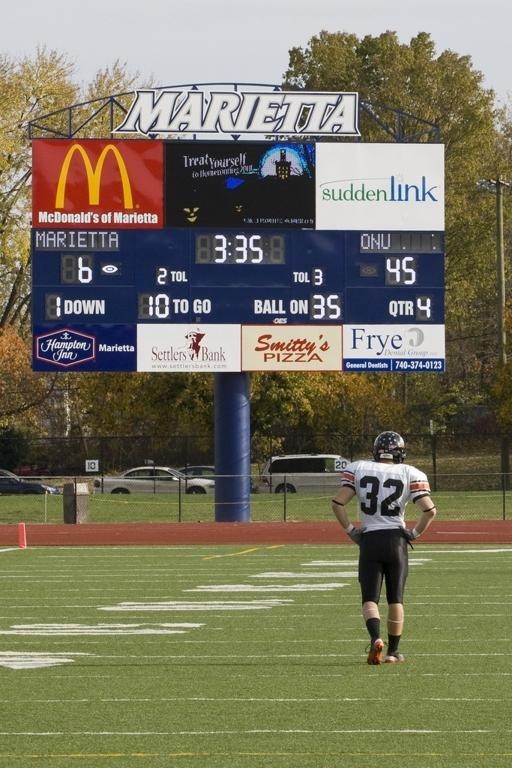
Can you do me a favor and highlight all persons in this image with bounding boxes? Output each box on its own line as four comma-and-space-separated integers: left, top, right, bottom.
332, 430, 436, 665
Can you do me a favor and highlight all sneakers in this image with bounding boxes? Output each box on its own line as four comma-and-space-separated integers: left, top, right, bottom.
384, 651, 405, 663
365, 638, 384, 665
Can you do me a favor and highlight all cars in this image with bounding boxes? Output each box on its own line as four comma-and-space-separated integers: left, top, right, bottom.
178, 467, 258, 492
0, 470, 61, 494
94, 464, 218, 495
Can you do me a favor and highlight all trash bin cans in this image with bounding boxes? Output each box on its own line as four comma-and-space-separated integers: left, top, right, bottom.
63, 483, 90, 524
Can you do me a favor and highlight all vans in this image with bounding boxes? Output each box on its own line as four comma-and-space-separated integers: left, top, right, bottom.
263, 451, 352, 493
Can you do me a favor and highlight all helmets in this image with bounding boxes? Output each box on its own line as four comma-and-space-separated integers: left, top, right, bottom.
373, 431, 406, 464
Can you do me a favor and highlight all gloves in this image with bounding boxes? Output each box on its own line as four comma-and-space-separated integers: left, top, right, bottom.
399, 525, 416, 542
347, 527, 367, 544
